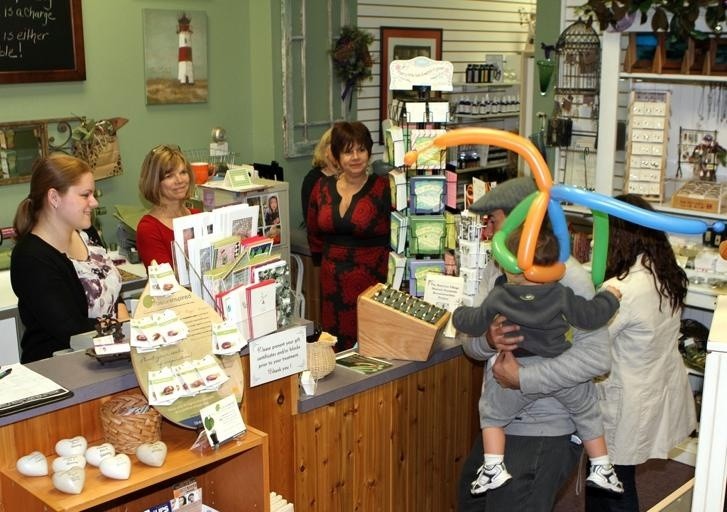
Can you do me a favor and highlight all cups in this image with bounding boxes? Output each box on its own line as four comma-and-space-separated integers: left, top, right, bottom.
190, 162, 216, 184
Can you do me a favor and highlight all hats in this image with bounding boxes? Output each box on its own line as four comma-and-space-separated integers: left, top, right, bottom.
466, 170, 540, 215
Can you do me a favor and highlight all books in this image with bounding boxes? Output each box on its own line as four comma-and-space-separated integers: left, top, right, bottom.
385, 99, 460, 298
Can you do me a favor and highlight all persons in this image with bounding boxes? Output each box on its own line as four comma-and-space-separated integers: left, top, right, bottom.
178, 495, 186, 506
306, 121, 392, 341
9, 154, 131, 364
450, 224, 625, 494
298, 126, 341, 268
264, 197, 280, 225
583, 193, 698, 512
135, 143, 204, 272
456, 176, 613, 511
187, 493, 195, 502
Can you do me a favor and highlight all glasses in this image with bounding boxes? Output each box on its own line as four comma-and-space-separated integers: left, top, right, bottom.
148, 143, 182, 154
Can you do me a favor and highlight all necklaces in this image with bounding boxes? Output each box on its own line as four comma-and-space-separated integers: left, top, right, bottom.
695, 83, 726, 125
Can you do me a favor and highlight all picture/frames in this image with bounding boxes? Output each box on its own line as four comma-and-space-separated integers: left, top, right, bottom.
380, 25, 442, 144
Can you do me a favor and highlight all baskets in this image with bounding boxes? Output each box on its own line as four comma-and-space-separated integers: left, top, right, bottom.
96, 389, 171, 454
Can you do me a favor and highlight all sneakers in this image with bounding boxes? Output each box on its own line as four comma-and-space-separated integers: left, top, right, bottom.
468, 462, 513, 495
583, 463, 624, 498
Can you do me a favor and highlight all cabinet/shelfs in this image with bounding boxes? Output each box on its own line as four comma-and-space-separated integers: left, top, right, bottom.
575, 0, 727, 467
0, 411, 271, 512
441, 81, 519, 211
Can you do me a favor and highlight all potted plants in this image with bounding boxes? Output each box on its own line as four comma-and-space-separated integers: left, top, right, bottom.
326, 25, 373, 80
